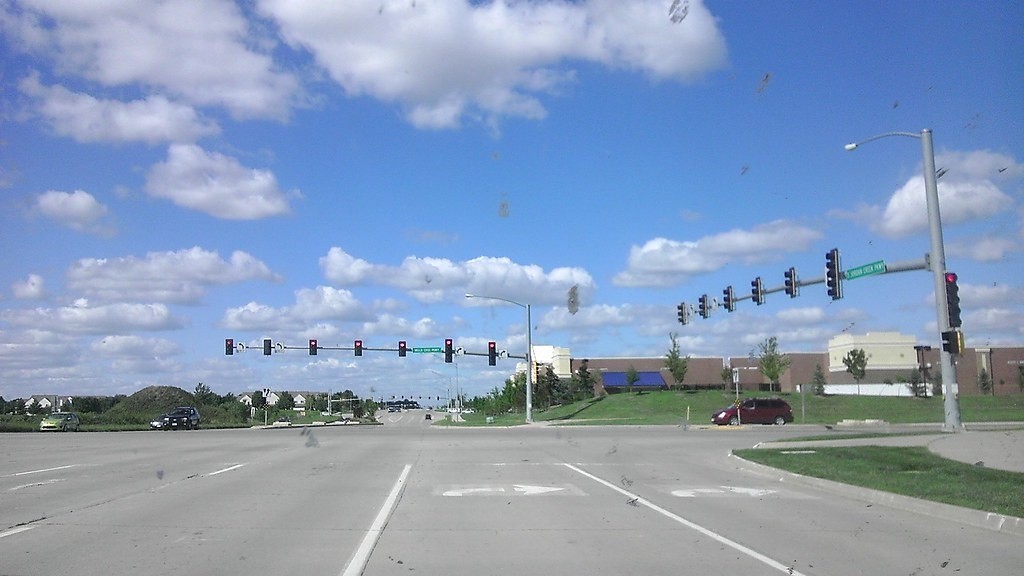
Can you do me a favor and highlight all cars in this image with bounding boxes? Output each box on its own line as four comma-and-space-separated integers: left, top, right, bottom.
149, 414, 169, 431
39, 411, 81, 433
388, 401, 419, 412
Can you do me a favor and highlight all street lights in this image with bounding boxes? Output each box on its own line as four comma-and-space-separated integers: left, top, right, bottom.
842, 129, 964, 432
911, 344, 932, 397
464, 292, 535, 423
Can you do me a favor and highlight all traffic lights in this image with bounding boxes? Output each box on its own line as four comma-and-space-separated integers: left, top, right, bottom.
444, 338, 454, 363
945, 271, 963, 328
751, 276, 762, 306
263, 338, 272, 355
354, 340, 362, 356
825, 248, 841, 300
677, 301, 687, 325
723, 286, 734, 312
225, 339, 234, 355
309, 339, 318, 355
488, 342, 496, 366
530, 361, 540, 384
941, 330, 964, 355
398, 341, 406, 357
784, 267, 797, 298
698, 295, 709, 319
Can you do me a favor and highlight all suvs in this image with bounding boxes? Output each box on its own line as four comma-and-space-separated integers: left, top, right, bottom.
163, 405, 202, 430
711, 396, 795, 426
